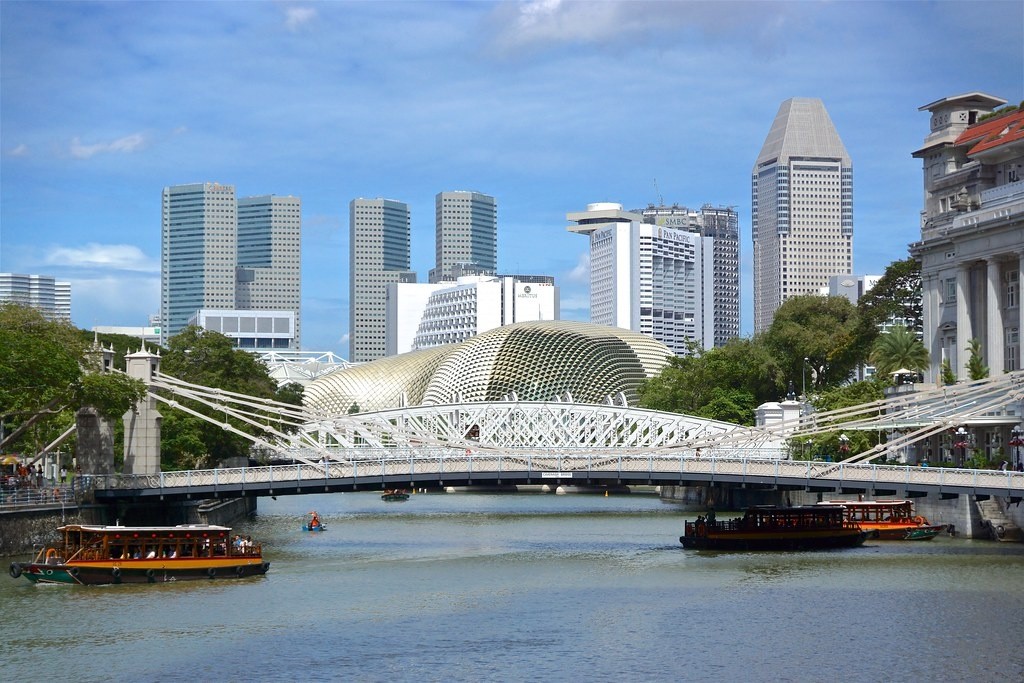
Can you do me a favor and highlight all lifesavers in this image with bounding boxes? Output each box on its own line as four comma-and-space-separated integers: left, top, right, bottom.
209, 568, 217, 576
46, 549, 58, 559
112, 568, 122, 578
914, 516, 924, 526
237, 567, 245, 574
8, 563, 21, 579
147, 569, 156, 578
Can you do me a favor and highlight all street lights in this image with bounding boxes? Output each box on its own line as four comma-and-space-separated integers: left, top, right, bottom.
838, 434, 849, 461
802, 357, 809, 415
955, 427, 968, 468
1012, 425, 1024, 471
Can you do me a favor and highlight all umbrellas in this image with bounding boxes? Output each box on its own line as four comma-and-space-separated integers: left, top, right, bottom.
888, 367, 916, 380
0, 454, 22, 465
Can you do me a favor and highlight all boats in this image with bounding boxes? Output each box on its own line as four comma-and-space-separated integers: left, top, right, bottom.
10, 524, 271, 585
814, 499, 948, 542
381, 491, 410, 502
679, 505, 880, 551
302, 511, 327, 532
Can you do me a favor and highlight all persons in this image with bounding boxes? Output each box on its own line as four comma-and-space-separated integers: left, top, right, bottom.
999, 460, 1024, 472
898, 373, 923, 385
694, 507, 715, 538
312, 517, 320, 527
77, 464, 80, 473
61, 464, 67, 483
732, 516, 755, 530
696, 448, 700, 461
18, 462, 42, 487
466, 449, 471, 457
119, 535, 258, 560
916, 459, 928, 467
384, 489, 405, 494
0, 465, 13, 484
839, 441, 849, 459
